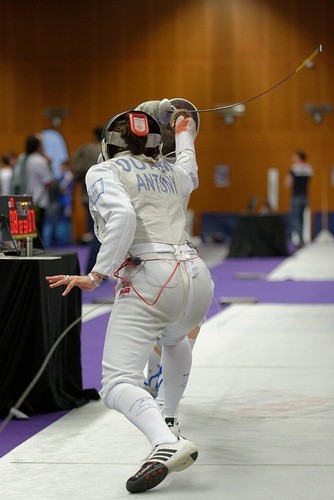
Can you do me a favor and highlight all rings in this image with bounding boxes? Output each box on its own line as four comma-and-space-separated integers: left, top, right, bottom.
63, 275, 71, 283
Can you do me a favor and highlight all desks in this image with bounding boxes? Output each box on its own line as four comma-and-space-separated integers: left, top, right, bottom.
0, 250, 102, 422
198, 213, 322, 258
226, 214, 294, 260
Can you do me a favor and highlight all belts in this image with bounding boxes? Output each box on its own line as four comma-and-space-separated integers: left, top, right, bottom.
124, 242, 197, 258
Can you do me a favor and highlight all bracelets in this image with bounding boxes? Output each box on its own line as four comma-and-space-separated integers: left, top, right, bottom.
87, 272, 102, 288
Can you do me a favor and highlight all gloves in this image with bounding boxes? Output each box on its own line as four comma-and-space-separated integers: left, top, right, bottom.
174, 116, 196, 154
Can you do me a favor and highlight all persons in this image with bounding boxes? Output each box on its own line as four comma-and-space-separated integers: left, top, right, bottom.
68, 125, 118, 277
284, 149, 315, 249
41, 106, 214, 492
0, 114, 71, 252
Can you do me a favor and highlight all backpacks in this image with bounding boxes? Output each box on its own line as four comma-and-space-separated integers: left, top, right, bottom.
10, 156, 29, 196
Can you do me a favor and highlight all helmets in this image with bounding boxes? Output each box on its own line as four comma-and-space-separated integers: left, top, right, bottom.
101, 109, 163, 162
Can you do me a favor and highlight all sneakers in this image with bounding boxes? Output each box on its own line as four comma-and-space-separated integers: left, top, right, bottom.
126, 435, 198, 493
164, 418, 179, 435
